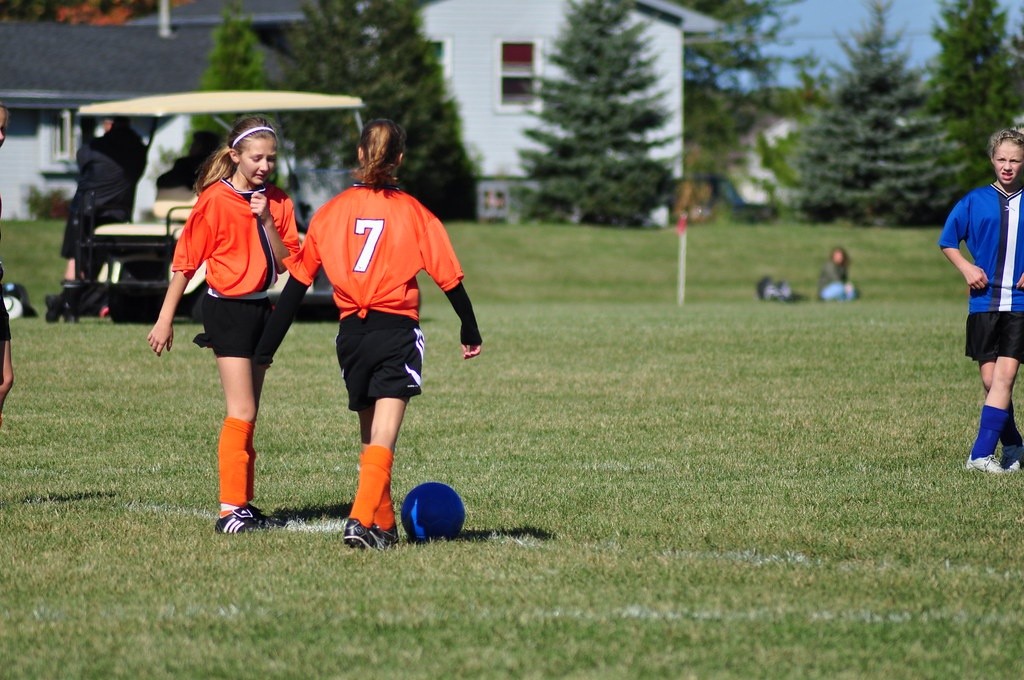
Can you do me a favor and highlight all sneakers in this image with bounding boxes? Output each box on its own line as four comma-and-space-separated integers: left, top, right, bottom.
247, 503, 288, 527
343, 518, 377, 551
215, 507, 260, 534
966, 455, 1004, 474
999, 442, 1024, 472
372, 522, 399, 545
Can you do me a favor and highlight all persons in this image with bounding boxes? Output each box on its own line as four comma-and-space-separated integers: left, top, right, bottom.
819, 246, 859, 301
156, 130, 221, 189
937, 125, 1023, 478
147, 115, 299, 536
254, 119, 481, 552
1, 100, 14, 416
46, 116, 142, 323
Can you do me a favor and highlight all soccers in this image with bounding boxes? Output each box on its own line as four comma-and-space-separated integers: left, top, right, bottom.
401, 482, 468, 543
